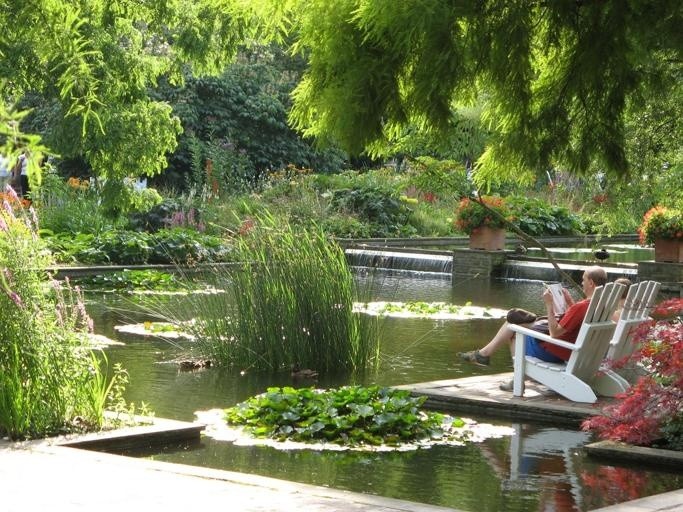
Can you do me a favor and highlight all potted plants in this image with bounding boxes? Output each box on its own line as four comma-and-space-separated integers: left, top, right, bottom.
468, 227, 506, 250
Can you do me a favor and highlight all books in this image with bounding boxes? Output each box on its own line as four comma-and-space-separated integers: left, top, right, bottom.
541, 280, 570, 316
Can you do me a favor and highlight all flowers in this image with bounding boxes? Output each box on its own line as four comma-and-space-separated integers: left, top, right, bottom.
637, 204, 683, 247
451, 195, 514, 240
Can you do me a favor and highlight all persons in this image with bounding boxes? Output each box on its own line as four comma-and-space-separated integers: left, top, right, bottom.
17, 148, 36, 201
612, 277, 633, 323
456, 265, 609, 392
0, 150, 17, 191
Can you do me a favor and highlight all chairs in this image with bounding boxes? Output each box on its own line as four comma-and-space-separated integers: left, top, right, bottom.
507, 280, 663, 406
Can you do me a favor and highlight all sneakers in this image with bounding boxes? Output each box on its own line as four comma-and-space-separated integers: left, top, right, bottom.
455, 348, 490, 368
500, 380, 526, 392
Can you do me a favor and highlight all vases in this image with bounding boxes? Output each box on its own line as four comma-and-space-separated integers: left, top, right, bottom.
652, 240, 682, 263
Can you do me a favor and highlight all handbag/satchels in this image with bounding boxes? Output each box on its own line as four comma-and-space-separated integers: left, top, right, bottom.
507, 308, 537, 324
